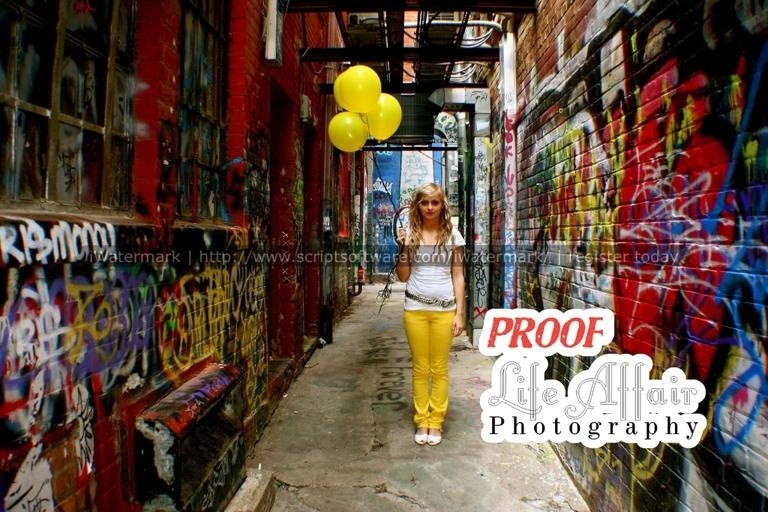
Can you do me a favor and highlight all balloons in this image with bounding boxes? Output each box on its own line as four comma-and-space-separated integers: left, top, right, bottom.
328, 112, 367, 152
331, 73, 351, 112
341, 65, 381, 112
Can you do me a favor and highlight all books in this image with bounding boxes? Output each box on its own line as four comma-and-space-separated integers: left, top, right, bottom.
367, 93, 403, 142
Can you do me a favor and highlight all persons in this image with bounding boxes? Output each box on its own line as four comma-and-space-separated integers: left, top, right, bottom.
395, 183, 467, 445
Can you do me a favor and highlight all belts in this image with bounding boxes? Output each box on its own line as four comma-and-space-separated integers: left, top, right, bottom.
404, 290, 454, 308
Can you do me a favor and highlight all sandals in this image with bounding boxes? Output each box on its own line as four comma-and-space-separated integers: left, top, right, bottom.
415, 433, 427, 445
428, 434, 442, 445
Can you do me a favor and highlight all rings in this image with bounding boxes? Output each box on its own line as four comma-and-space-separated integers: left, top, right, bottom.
460, 330, 462, 332
456, 330, 459, 332
